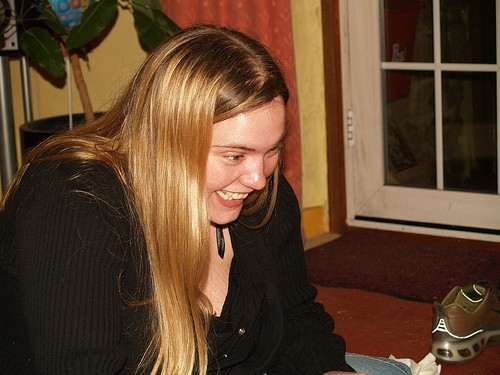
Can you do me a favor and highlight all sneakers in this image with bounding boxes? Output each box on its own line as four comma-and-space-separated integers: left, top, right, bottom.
431, 280, 499, 363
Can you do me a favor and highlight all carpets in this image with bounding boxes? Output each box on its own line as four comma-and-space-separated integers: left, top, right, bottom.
304, 233, 499, 305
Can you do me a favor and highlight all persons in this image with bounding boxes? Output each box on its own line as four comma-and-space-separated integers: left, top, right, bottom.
0, 23, 365, 375
